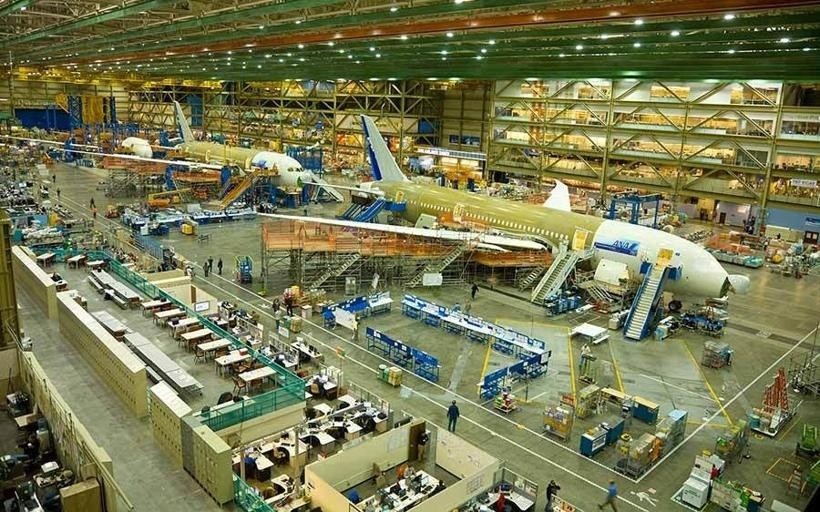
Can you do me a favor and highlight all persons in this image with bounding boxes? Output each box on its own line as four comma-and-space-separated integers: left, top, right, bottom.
544, 479, 619, 512
4, 167, 223, 279
222, 292, 368, 430
15, 434, 75, 512
260, 201, 307, 216
740, 225, 781, 252
579, 344, 591, 368
454, 282, 479, 313
372, 400, 459, 479
700, 210, 717, 224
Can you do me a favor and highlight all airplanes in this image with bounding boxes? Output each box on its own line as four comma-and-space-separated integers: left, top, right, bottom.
304, 115, 751, 300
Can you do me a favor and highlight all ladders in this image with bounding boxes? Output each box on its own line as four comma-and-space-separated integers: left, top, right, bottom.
764, 366, 788, 416
785, 467, 803, 500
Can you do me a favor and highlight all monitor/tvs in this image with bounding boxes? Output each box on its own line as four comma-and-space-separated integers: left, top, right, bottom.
366, 474, 432, 510
247, 401, 372, 453
477, 491, 489, 503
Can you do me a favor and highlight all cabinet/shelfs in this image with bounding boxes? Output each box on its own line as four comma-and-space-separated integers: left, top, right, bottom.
543, 383, 688, 478
